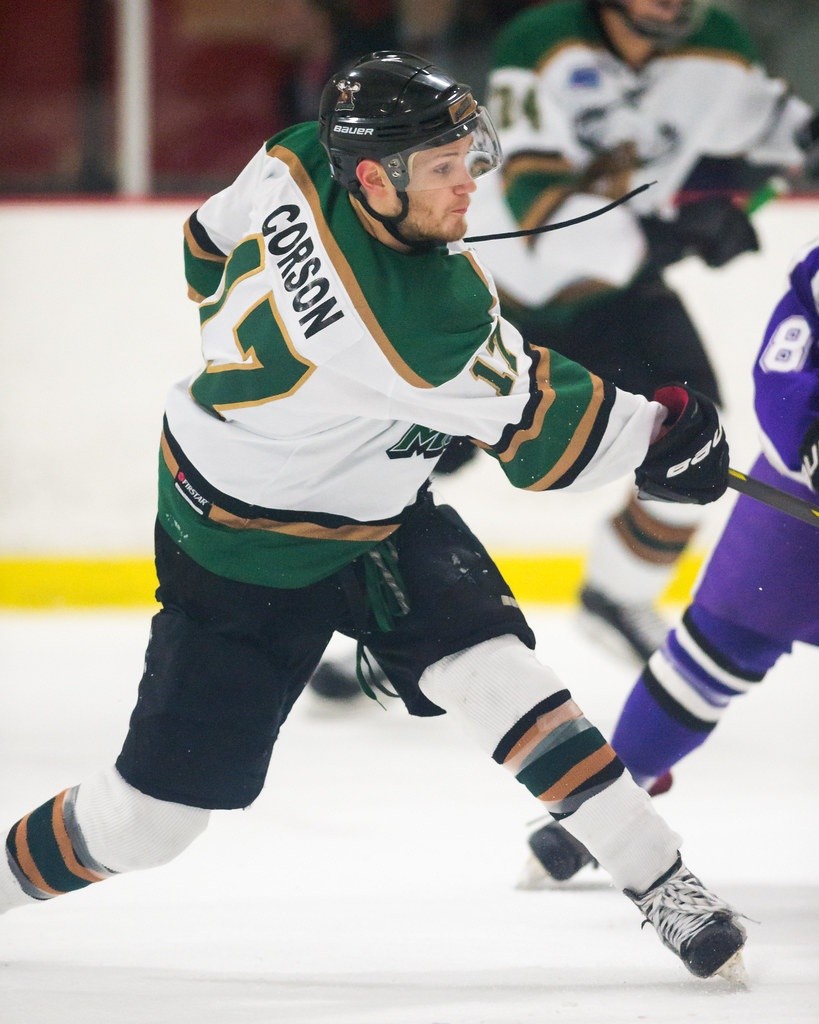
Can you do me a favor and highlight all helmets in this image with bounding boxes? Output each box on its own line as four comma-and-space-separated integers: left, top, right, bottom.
318, 50, 486, 188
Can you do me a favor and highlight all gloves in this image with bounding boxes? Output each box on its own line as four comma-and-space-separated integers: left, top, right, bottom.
634, 383, 730, 505
678, 196, 760, 269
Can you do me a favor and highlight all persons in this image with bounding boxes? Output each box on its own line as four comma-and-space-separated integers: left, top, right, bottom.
0, 48, 745, 978
299, 1, 819, 707
526, 247, 818, 881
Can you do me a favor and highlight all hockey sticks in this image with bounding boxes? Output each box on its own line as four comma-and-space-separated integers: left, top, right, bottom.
725, 467, 819, 530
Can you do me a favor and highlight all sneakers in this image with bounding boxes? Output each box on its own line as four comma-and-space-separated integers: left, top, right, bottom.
311, 648, 389, 700
623, 849, 759, 993
579, 585, 675, 671
529, 819, 599, 882
